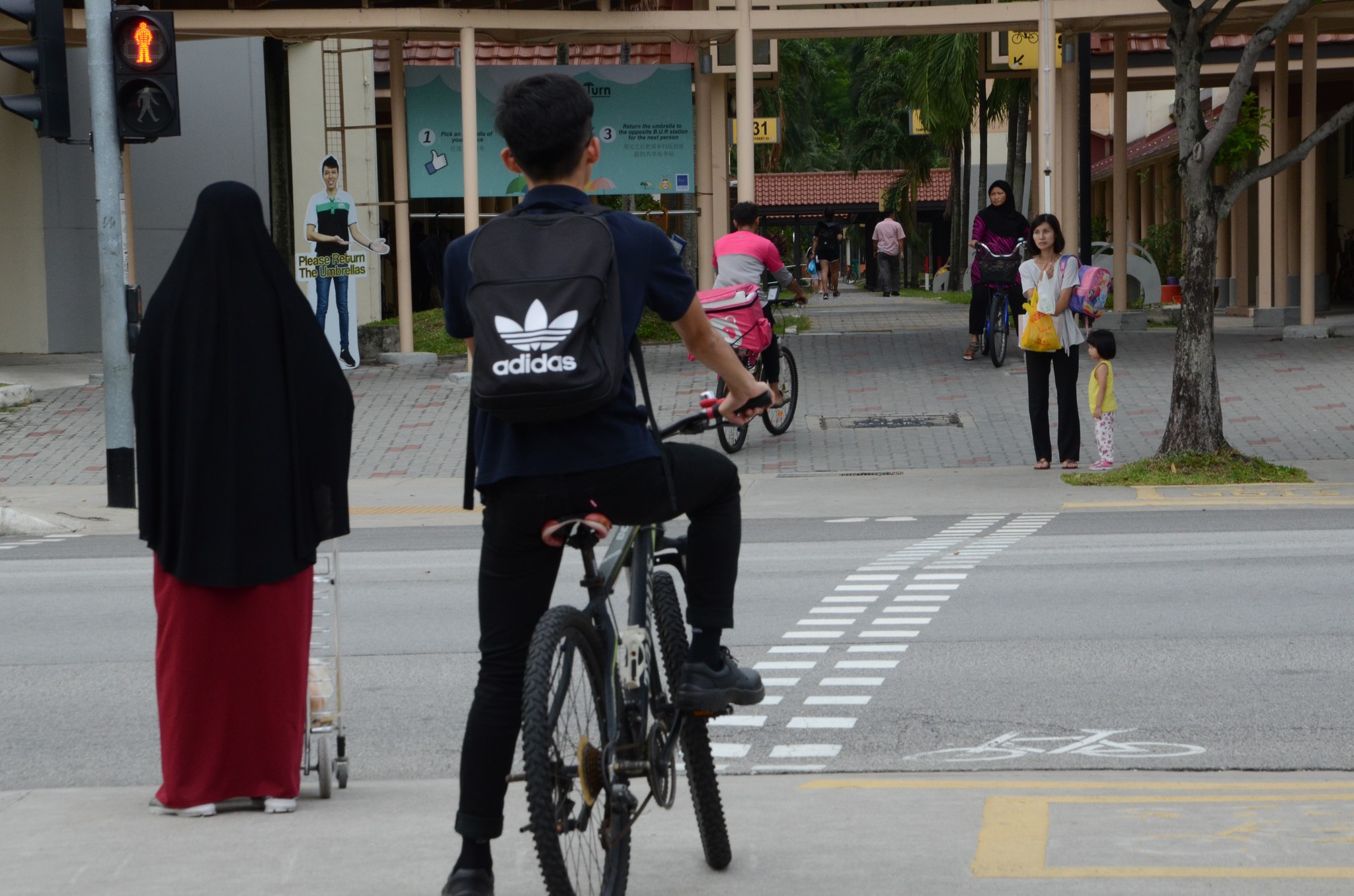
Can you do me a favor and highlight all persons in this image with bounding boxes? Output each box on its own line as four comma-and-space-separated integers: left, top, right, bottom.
711, 201, 807, 407
304, 155, 390, 367
1018, 213, 1085, 469
130, 179, 356, 818
807, 205, 843, 299
1086, 328, 1117, 470
444, 74, 776, 896
1167, 272, 1185, 293
963, 180, 1031, 360
872, 206, 906, 297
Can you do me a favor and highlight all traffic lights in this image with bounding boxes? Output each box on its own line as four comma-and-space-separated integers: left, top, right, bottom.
1, 1, 75, 139
112, 8, 185, 140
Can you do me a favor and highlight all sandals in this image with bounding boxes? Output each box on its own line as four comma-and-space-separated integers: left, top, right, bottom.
1089, 459, 1113, 471
963, 345, 979, 361
1034, 460, 1050, 469
1061, 459, 1078, 469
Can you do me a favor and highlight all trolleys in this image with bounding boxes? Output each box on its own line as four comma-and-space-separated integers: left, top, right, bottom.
288, 538, 353, 799
496, 392, 772, 890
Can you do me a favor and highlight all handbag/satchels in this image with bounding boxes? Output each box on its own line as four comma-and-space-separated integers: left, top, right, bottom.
1036, 270, 1056, 315
1018, 312, 1063, 352
1021, 287, 1061, 352
807, 259, 817, 275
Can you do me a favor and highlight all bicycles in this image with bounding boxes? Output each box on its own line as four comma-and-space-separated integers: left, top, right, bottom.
971, 238, 1034, 366
843, 260, 862, 284
713, 296, 811, 455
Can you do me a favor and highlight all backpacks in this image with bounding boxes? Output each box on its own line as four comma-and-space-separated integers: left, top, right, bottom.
1059, 254, 1111, 319
815, 221, 840, 256
465, 204, 637, 422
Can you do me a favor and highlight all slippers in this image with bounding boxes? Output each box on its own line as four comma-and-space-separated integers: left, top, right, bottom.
770, 388, 791, 408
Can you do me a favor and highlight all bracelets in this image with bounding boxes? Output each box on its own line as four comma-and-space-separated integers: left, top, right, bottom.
368, 242, 372, 249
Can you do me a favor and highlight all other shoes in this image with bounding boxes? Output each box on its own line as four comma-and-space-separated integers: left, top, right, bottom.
149, 796, 216, 818
441, 867, 495, 895
676, 645, 765, 711
823, 292, 829, 299
883, 292, 890, 297
891, 291, 900, 296
338, 348, 356, 367
833, 290, 840, 298
262, 795, 299, 813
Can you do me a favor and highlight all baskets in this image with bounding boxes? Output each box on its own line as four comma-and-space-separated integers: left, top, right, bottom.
977, 251, 1022, 285
